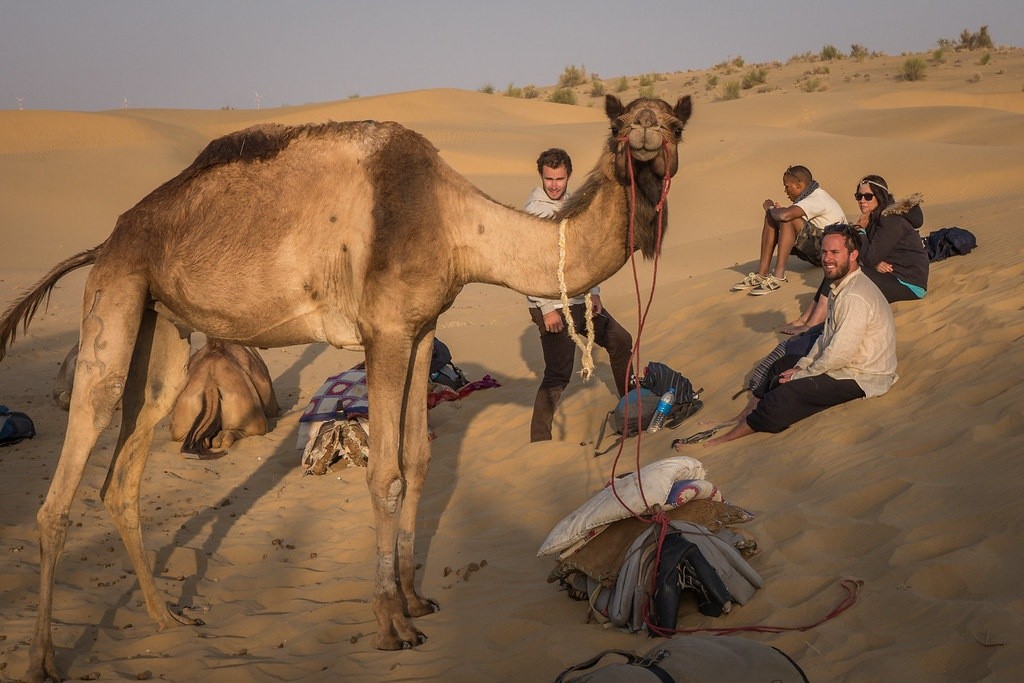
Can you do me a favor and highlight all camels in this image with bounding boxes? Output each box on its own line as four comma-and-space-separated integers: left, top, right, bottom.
0, 94, 694, 682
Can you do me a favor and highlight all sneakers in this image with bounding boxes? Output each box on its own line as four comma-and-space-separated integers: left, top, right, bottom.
750, 276, 788, 295
734, 274, 770, 289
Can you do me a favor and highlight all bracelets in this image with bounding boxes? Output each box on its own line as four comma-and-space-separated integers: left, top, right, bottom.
857, 227, 867, 234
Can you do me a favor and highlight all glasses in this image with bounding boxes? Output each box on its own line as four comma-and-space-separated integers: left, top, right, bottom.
825, 224, 858, 250
785, 165, 800, 182
854, 193, 875, 201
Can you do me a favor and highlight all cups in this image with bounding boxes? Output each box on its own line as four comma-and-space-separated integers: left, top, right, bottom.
646, 388, 675, 434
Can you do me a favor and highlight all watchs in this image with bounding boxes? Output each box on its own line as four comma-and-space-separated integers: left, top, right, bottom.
768, 205, 775, 213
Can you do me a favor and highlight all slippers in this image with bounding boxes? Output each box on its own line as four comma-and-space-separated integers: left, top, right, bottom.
664, 400, 703, 429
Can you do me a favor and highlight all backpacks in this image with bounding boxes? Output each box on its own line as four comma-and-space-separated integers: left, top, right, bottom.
594, 387, 663, 455
430, 337, 468, 391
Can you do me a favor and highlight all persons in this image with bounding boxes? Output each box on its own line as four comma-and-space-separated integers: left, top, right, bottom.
672, 224, 899, 451
524, 149, 637, 444
731, 165, 848, 295
780, 175, 930, 335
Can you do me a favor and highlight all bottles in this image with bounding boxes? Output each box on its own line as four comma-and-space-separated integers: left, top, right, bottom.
431, 370, 439, 379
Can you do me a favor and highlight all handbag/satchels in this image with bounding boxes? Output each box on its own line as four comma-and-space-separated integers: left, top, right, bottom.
639, 361, 694, 402
749, 340, 786, 391
554, 636, 809, 683
